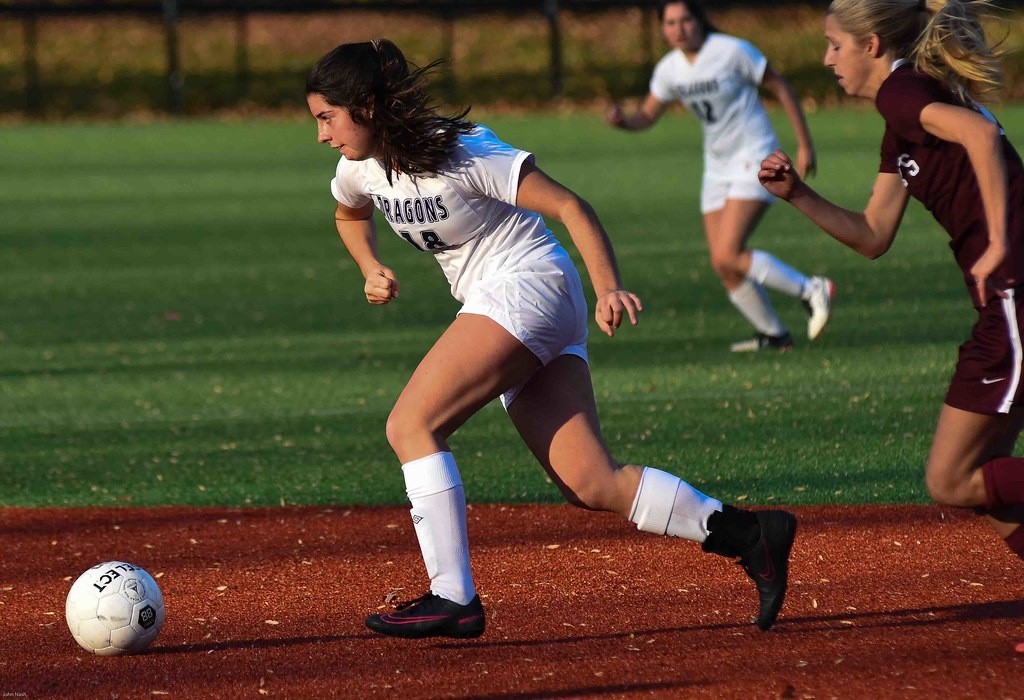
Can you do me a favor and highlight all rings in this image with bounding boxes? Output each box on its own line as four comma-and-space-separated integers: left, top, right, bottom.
974, 278, 978, 283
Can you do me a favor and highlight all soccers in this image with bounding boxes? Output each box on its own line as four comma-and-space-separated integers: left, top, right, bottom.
65, 561, 165, 657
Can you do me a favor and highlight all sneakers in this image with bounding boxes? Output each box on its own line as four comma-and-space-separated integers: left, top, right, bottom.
736, 509, 797, 631
731, 332, 794, 351
801, 276, 834, 340
364, 590, 485, 639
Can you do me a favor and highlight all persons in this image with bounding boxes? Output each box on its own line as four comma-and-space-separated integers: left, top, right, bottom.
757, 0, 1024, 652
602, 0, 836, 353
303, 36, 797, 637
